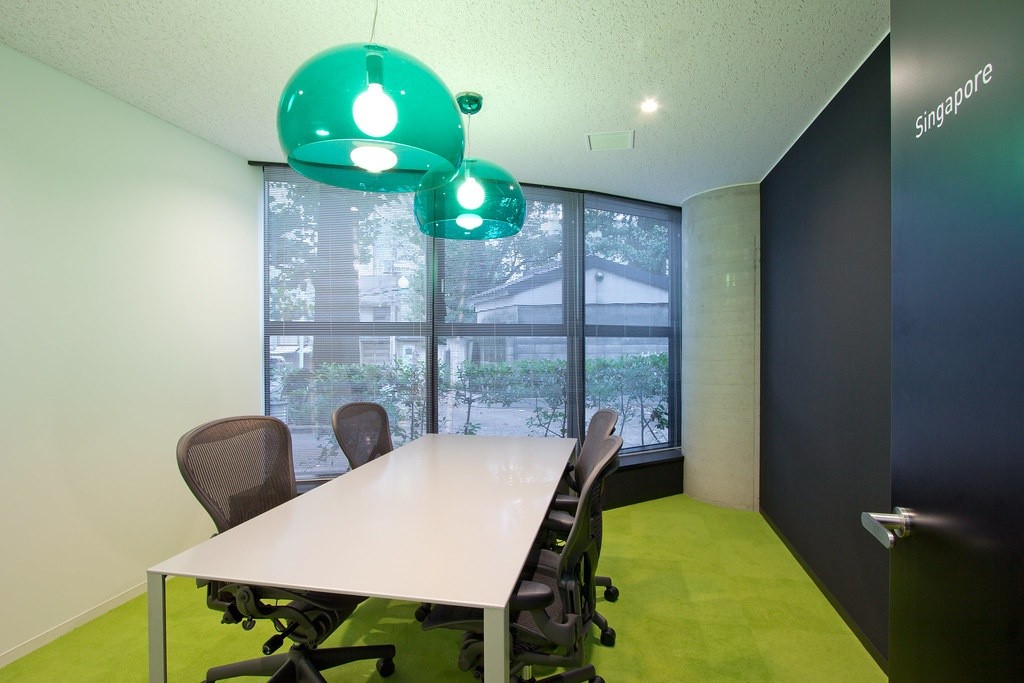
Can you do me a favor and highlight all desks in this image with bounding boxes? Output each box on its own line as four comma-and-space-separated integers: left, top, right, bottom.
145, 433, 578, 683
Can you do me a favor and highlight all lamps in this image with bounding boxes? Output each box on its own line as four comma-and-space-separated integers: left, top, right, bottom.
413, 92, 527, 241
276, 0, 466, 193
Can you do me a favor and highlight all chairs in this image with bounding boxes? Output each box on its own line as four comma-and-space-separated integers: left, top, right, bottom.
332, 402, 393, 470
176, 416, 398, 683
542, 406, 620, 647
420, 435, 625, 683
